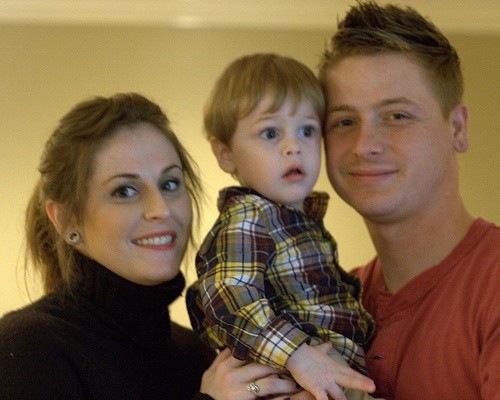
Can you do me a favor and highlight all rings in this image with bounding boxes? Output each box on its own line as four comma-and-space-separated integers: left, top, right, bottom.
246, 382, 259, 396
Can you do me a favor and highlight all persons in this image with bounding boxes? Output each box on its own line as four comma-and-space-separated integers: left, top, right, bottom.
316, 0, 500, 400
0, 92, 303, 400
185, 52, 377, 400
